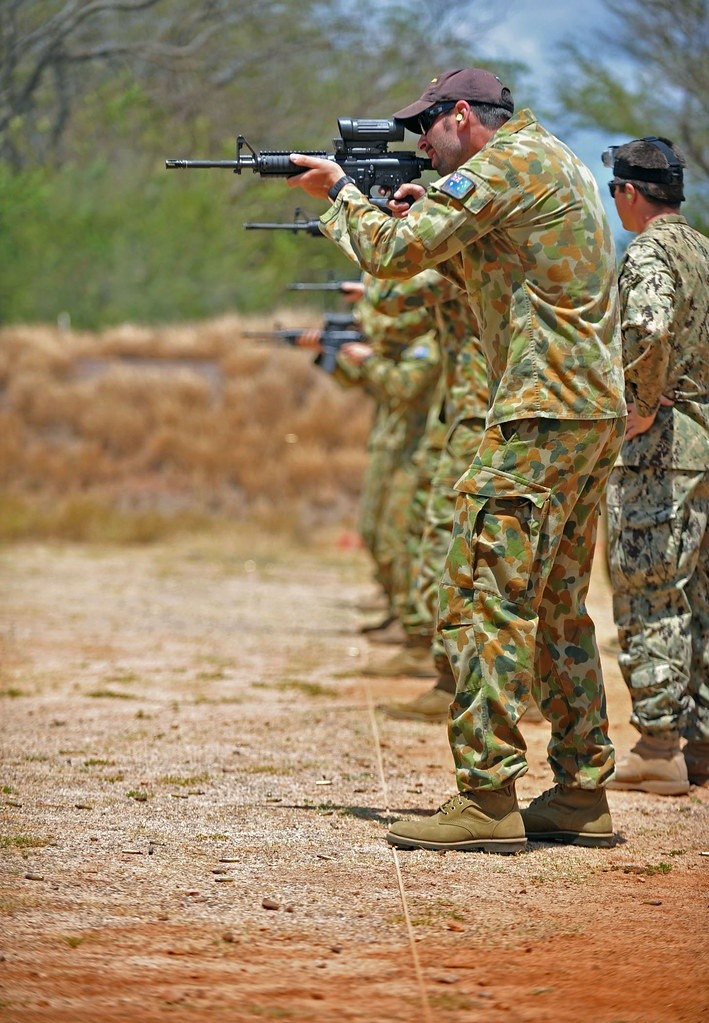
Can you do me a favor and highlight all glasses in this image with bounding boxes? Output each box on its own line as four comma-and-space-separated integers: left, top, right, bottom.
419, 100, 473, 137
608, 180, 644, 198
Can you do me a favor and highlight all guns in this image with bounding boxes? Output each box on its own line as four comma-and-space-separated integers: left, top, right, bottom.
286, 270, 359, 294
241, 312, 363, 372
245, 198, 390, 238
166, 118, 433, 216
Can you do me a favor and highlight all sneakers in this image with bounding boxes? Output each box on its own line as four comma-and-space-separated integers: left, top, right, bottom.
518, 782, 617, 847
681, 736, 709, 785
600, 732, 690, 794
522, 694, 542, 721
386, 784, 528, 854
360, 616, 406, 644
380, 680, 455, 722
361, 632, 437, 676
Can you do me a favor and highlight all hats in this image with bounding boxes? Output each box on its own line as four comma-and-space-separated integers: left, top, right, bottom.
601, 136, 686, 202
392, 67, 515, 135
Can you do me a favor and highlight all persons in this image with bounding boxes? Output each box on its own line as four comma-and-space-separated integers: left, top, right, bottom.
599, 136, 709, 797
285, 70, 626, 854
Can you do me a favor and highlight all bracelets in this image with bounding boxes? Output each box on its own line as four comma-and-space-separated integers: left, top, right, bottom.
327, 175, 355, 206
